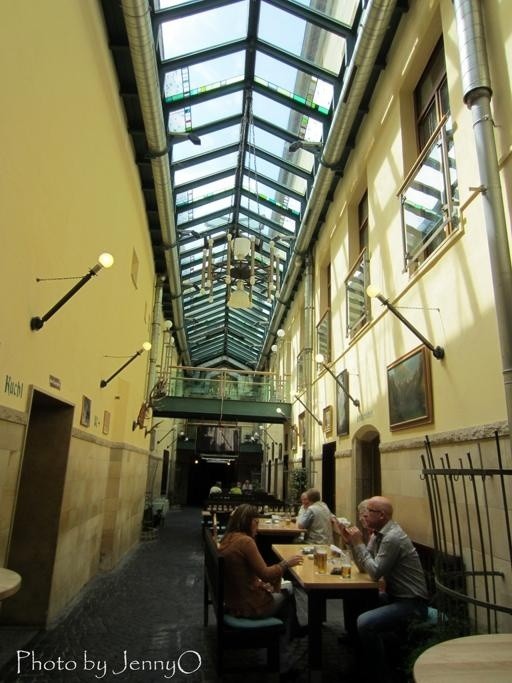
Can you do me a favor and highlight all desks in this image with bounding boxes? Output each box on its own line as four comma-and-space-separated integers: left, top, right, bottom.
412, 633, 512, 683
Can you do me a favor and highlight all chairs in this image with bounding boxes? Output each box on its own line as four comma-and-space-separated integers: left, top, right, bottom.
200, 486, 346, 545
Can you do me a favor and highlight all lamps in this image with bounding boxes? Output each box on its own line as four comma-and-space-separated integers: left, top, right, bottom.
293, 393, 326, 428
144, 415, 196, 455
313, 351, 363, 412
271, 408, 303, 440
28, 254, 116, 333
98, 340, 160, 390
200, 103, 280, 309
245, 426, 277, 450
364, 286, 449, 360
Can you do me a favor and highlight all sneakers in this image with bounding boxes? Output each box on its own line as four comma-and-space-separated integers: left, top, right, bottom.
295, 623, 309, 640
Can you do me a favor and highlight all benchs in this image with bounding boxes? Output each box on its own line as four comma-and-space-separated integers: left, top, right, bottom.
380, 530, 472, 643
200, 526, 289, 676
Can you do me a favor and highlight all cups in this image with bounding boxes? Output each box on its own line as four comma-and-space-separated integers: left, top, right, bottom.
262, 513, 292, 527
301, 542, 352, 577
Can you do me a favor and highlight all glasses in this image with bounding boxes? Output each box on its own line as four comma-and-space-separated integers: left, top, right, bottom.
365, 507, 381, 514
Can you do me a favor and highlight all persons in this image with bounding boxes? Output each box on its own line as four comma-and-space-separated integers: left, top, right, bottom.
243, 479, 254, 491
216, 502, 310, 639
293, 491, 348, 550
297, 488, 333, 546
339, 497, 386, 647
333, 496, 428, 673
229, 481, 244, 494
209, 481, 223, 496
